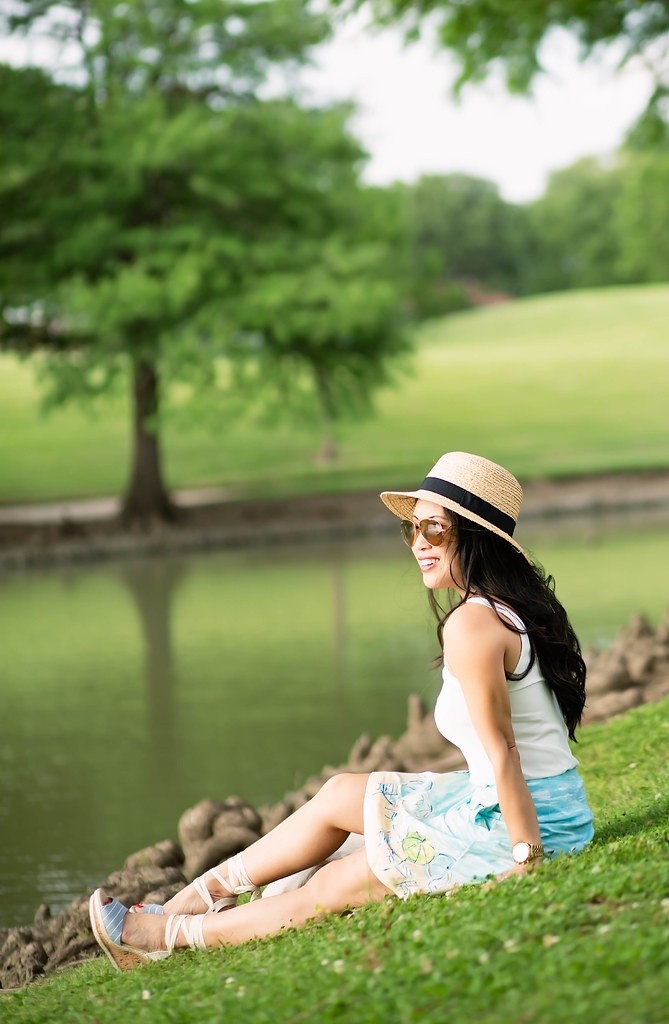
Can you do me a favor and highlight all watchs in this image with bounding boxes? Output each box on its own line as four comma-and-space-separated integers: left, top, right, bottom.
512, 842, 543, 865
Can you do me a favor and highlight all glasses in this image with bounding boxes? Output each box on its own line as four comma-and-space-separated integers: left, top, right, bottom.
399, 518, 455, 548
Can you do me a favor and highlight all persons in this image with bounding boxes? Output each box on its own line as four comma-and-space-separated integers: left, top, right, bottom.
86, 450, 619, 974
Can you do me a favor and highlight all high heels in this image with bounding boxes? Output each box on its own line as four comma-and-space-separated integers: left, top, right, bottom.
89, 888, 207, 974
129, 852, 262, 915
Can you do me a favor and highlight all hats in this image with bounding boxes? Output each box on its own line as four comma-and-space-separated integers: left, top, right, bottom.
380, 452, 530, 564
